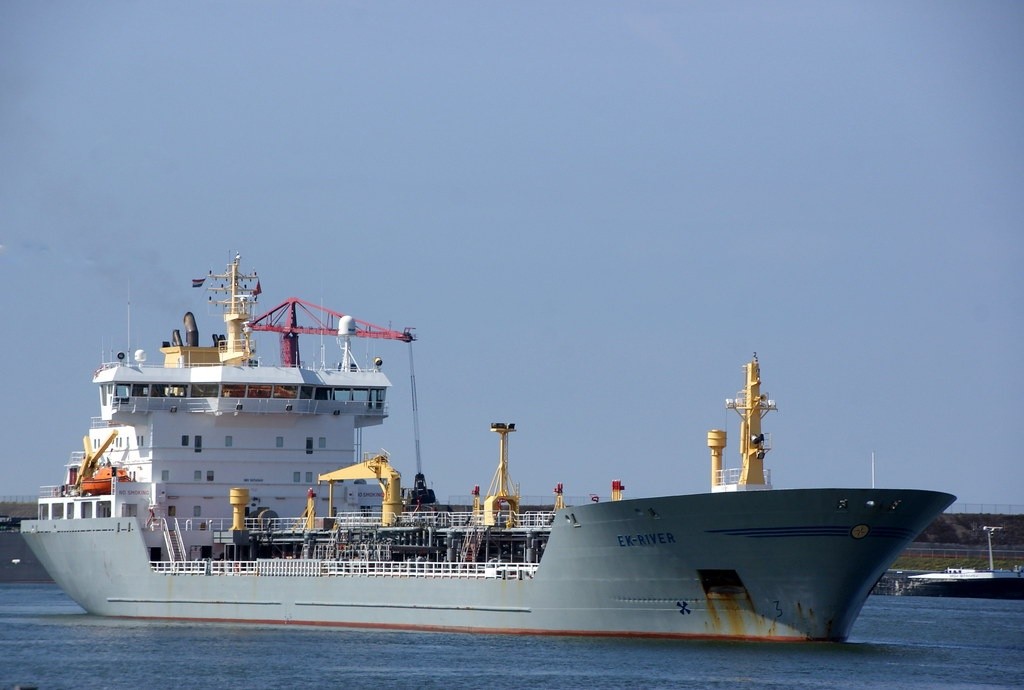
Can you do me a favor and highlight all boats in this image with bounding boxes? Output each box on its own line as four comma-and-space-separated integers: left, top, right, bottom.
20, 250, 957, 643
80, 477, 129, 495
907, 526, 1024, 583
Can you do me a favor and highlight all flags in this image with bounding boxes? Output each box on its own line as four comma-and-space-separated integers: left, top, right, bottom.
192, 278, 205, 287
